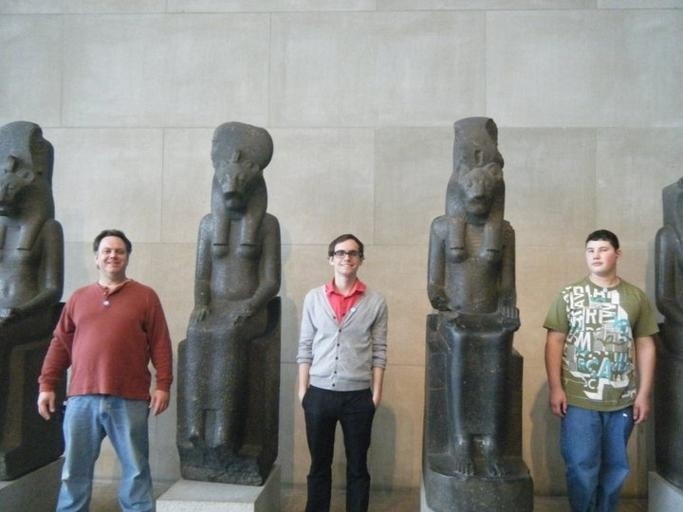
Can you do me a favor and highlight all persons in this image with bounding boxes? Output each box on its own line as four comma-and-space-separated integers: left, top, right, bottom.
34, 227, 174, 512
541, 229, 661, 512
294, 234, 388, 511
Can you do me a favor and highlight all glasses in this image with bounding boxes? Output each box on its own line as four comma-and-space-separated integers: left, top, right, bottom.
334, 250, 360, 255
